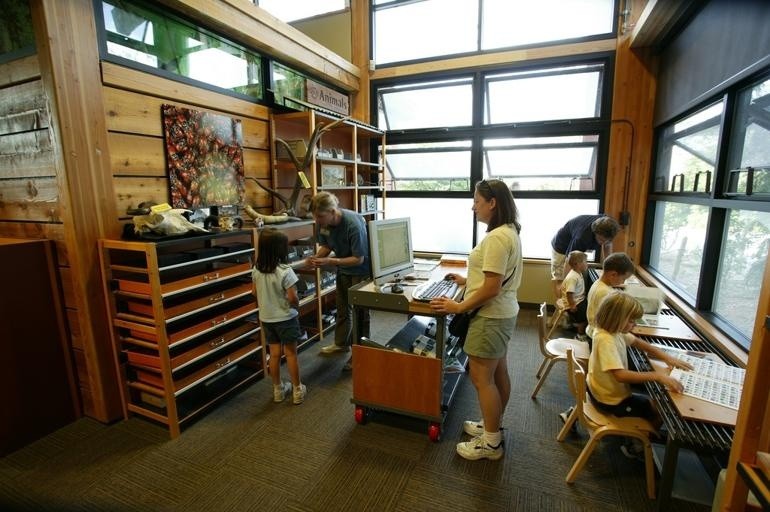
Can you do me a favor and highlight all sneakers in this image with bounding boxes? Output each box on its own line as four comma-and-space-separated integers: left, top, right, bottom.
293, 385, 307, 404
274, 382, 292, 403
320, 344, 351, 354
621, 443, 644, 461
455, 435, 503, 461
464, 419, 506, 441
559, 406, 577, 433
574, 333, 587, 342
343, 355, 352, 370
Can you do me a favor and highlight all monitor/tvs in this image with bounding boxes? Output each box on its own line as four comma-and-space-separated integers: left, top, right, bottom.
369, 217, 417, 294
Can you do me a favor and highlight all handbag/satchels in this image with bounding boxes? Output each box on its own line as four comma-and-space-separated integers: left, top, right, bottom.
449, 312, 469, 337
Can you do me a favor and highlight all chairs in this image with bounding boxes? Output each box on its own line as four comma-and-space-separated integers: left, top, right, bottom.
525, 300, 589, 399
544, 270, 582, 337
556, 346, 662, 502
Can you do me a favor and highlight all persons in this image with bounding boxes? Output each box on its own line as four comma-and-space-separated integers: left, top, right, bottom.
429, 178, 523, 460
560, 251, 589, 342
250, 227, 307, 406
550, 214, 619, 300
585, 290, 694, 460
586, 252, 635, 353
306, 190, 372, 373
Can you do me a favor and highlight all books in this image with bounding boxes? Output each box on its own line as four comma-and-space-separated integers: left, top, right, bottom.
665, 353, 746, 412
440, 255, 467, 264
621, 284, 669, 329
321, 305, 337, 323
409, 314, 467, 374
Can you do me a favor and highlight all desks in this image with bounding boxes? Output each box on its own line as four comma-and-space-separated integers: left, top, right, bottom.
588, 260, 748, 512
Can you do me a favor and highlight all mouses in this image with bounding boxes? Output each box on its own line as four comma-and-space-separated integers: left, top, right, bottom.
444, 275, 455, 280
392, 283, 403, 293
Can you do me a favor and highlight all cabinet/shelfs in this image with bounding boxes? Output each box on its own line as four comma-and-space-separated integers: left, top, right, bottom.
347, 255, 472, 443
97, 222, 267, 440
1, 231, 87, 459
267, 108, 386, 230
258, 217, 339, 362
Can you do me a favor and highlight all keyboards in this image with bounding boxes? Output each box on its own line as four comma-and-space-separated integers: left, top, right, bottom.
415, 280, 458, 301
